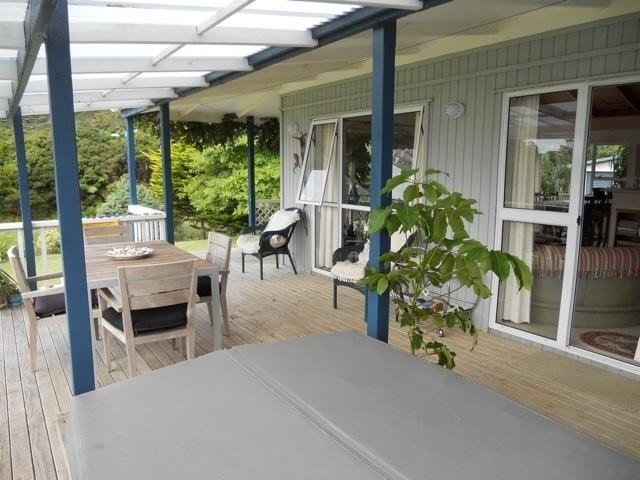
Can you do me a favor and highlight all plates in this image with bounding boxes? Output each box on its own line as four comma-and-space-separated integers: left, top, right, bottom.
105, 245, 155, 261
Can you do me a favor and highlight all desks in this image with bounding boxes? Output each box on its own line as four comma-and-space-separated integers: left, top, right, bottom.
84, 239, 223, 361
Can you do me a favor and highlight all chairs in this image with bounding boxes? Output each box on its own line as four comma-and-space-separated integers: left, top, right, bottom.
533, 186, 640, 247
328, 208, 418, 323
82, 222, 136, 244
196, 229, 235, 338
236, 206, 303, 281
97, 256, 200, 377
7, 243, 104, 374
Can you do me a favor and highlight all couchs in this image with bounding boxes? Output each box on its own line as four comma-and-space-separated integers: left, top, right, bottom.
531, 243, 640, 328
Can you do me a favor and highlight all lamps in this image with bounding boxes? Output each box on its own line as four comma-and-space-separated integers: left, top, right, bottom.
443, 100, 467, 121
